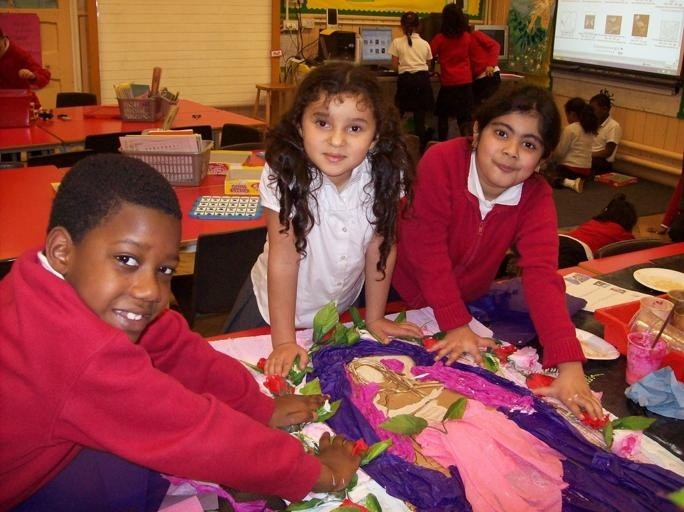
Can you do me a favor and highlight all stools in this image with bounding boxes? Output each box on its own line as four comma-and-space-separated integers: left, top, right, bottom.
254, 84, 295, 127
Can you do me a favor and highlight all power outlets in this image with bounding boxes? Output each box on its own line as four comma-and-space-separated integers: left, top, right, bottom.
284, 19, 298, 32
302, 17, 315, 27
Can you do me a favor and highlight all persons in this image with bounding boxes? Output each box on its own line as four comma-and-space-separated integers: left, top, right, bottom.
388, 11, 435, 127
463, 11, 501, 106
220, 64, 424, 376
430, 3, 473, 141
539, 97, 599, 194
0, 26, 51, 113
589, 87, 622, 175
495, 192, 638, 277
360, 83, 607, 423
646, 154, 684, 243
0, 153, 360, 512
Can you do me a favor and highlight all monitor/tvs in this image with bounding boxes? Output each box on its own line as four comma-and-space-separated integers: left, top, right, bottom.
361, 25, 393, 76
474, 24, 508, 60
550, 0, 684, 86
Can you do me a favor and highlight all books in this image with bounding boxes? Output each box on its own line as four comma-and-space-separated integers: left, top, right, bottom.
594, 172, 639, 187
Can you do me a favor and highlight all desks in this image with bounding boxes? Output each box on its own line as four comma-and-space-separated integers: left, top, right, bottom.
196, 266, 684, 512
62, 154, 270, 247
582, 239, 684, 304
0, 165, 63, 264
0, 125, 62, 154
35, 95, 266, 138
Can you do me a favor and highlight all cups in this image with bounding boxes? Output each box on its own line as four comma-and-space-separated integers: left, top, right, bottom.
625, 331, 667, 386
640, 295, 675, 322
667, 291, 684, 308
625, 306, 683, 353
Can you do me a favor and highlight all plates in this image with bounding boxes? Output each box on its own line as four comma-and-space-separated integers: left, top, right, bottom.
575, 327, 621, 361
633, 267, 684, 294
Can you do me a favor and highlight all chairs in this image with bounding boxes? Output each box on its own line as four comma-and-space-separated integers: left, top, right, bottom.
558, 232, 593, 272
178, 223, 270, 336
86, 130, 148, 152
598, 240, 667, 257
169, 125, 216, 140
58, 88, 96, 105
218, 123, 267, 148
